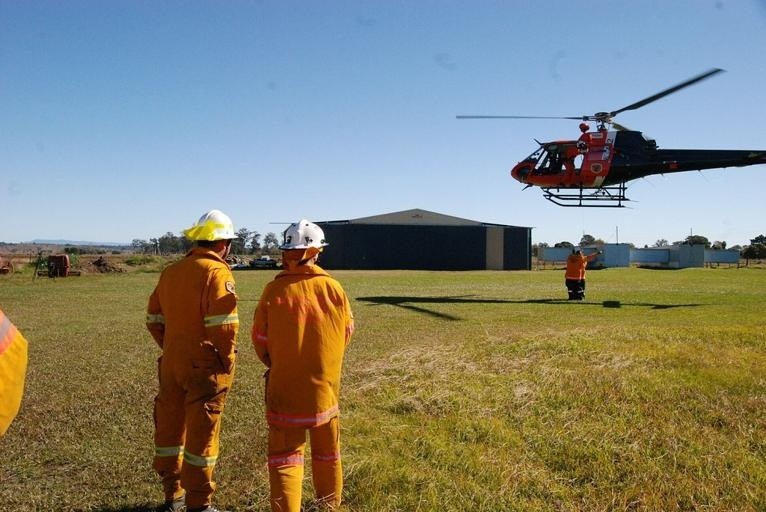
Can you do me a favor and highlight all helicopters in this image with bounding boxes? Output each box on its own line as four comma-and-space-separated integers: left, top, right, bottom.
455, 68, 766, 208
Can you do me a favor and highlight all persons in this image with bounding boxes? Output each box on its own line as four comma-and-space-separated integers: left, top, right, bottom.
565, 245, 603, 299
144, 206, 240, 512
251, 218, 355, 512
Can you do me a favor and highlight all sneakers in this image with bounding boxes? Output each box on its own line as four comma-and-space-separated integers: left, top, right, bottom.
166, 496, 187, 512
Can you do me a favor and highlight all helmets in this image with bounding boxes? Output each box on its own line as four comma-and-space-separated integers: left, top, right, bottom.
277, 218, 330, 251
182, 208, 239, 241
